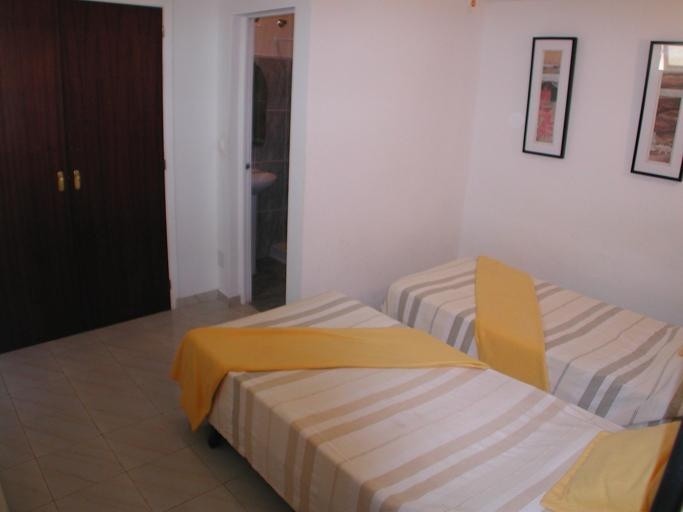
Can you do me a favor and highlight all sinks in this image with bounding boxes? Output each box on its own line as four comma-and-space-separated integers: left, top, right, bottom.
251, 162, 279, 194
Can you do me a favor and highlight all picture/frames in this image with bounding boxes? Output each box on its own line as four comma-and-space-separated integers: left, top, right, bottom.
522, 36, 578, 158
631, 40, 683, 181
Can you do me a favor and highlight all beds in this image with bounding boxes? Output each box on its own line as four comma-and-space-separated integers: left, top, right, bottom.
170, 291, 683, 512
383, 254, 683, 428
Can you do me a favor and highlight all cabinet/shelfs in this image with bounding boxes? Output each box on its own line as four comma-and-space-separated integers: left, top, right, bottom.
0, 0, 170, 356
